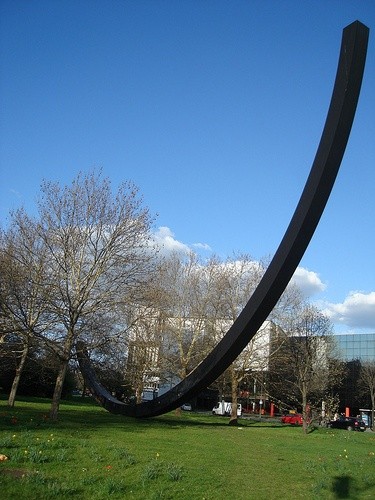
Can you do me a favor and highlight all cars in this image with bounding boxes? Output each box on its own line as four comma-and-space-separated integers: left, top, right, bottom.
326, 417, 366, 432
281, 413, 312, 426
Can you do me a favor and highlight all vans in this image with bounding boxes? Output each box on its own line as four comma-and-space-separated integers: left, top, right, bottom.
181, 403, 192, 412
212, 401, 242, 416
